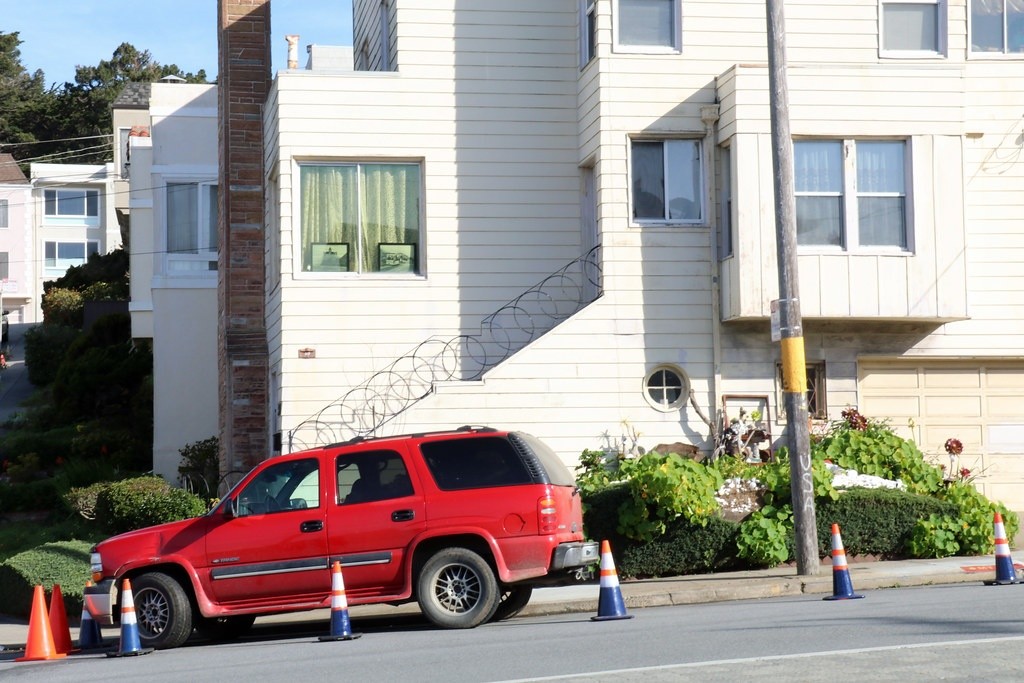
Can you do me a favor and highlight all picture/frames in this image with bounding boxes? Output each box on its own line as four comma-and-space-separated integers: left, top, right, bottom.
310, 242, 350, 272
378, 242, 418, 274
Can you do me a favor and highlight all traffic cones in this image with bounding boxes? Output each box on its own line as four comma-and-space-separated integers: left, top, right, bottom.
75, 580, 115, 649
49, 584, 76, 653
104, 579, 155, 656
982, 512, 1023, 586
15, 585, 66, 662
821, 524, 864, 600
317, 561, 361, 641
591, 540, 634, 620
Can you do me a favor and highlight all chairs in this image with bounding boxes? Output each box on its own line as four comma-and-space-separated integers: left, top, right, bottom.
341, 471, 415, 506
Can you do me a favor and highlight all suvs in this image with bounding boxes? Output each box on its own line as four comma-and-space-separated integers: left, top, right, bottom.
84, 426, 599, 642
